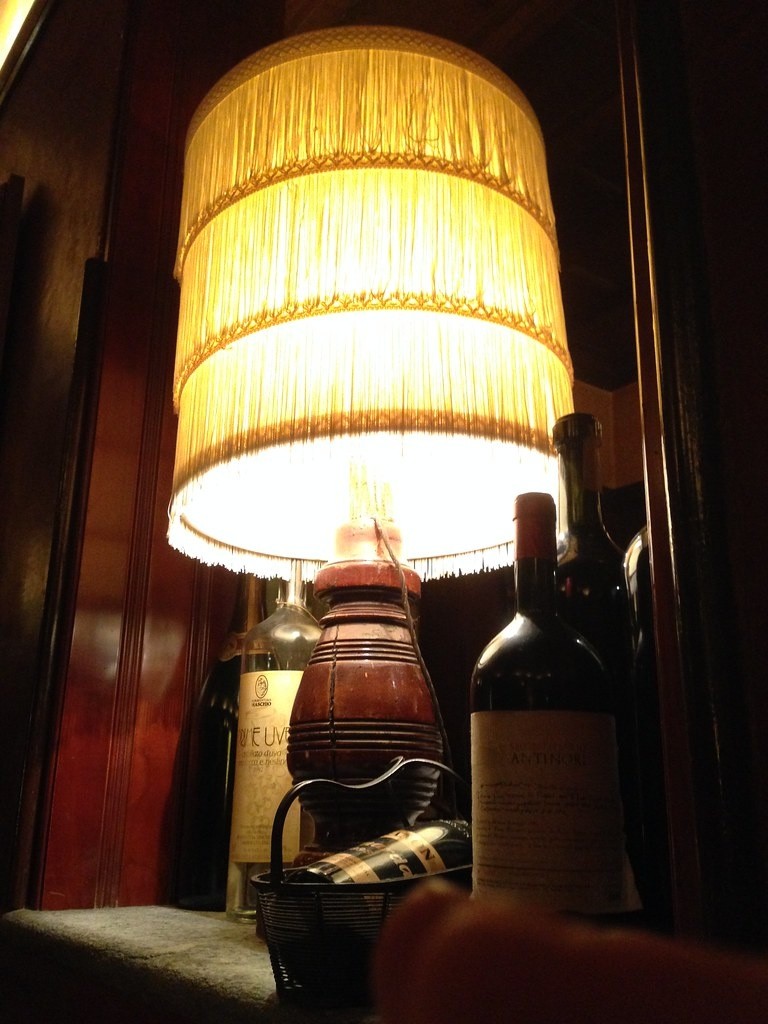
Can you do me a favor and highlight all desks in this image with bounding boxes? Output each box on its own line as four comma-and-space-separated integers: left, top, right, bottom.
0, 905, 284, 1024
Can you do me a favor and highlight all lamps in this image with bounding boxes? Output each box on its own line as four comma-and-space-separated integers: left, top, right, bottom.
165, 25, 574, 944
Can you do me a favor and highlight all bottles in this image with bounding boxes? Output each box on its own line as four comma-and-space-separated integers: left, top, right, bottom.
469, 491, 626, 922
552, 413, 634, 706
224, 560, 324, 923
301, 815, 472, 883
172, 571, 267, 914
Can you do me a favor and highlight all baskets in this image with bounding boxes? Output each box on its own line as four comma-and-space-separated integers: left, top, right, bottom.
250, 760, 471, 1014
